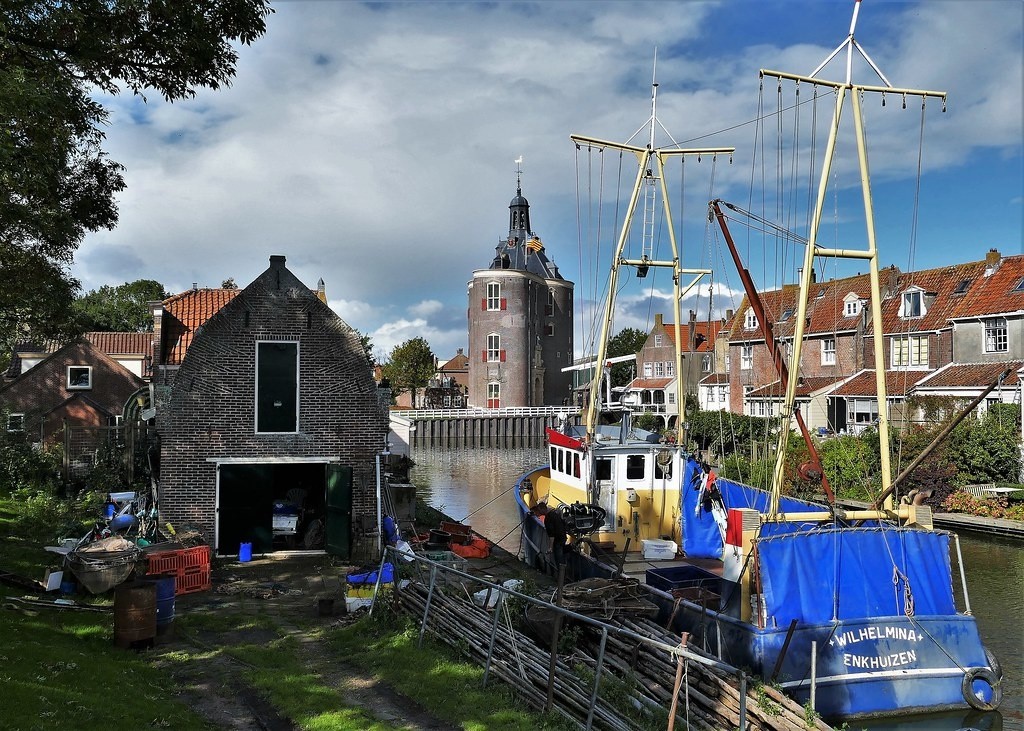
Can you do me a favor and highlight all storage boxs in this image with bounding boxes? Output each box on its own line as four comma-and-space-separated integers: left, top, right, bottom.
272, 514, 298, 531
416, 551, 468, 589
474, 579, 523, 608
640, 539, 676, 559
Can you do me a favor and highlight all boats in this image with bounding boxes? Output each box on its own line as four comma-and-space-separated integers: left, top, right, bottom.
514, 0, 1006, 731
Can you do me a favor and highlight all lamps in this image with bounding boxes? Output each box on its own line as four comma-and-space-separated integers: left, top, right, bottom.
137, 391, 147, 413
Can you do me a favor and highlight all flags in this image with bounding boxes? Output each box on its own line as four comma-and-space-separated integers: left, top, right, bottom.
527, 231, 542, 252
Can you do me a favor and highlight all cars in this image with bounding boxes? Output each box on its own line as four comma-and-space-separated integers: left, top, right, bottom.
73, 454, 103, 467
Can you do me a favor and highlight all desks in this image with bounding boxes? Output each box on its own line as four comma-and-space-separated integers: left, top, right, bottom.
982, 487, 1024, 493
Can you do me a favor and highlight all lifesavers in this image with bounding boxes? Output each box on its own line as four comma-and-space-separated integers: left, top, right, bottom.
981, 646, 1004, 679
959, 666, 1006, 713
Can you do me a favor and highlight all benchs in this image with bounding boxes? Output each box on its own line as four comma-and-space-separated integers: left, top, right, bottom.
959, 482, 998, 500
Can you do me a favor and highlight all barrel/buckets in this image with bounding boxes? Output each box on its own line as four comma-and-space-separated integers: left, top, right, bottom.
429, 529, 451, 542
115, 582, 158, 641
136, 574, 175, 624
